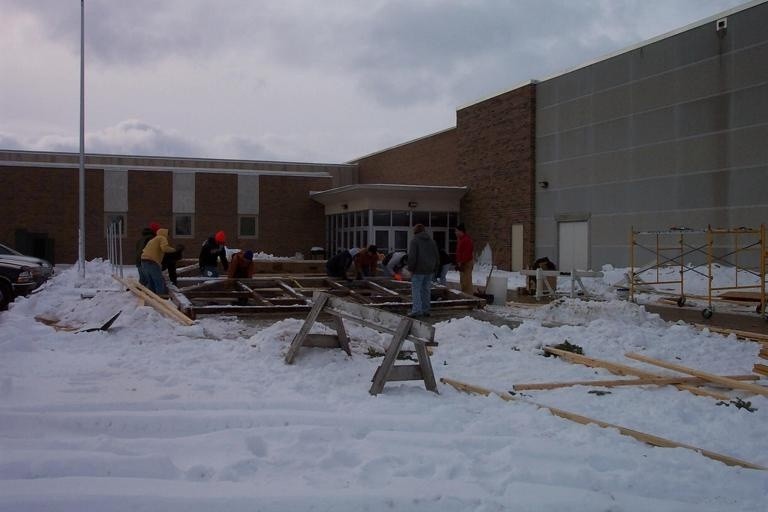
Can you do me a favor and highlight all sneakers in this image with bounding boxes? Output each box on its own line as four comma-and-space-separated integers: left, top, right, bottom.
407, 311, 423, 318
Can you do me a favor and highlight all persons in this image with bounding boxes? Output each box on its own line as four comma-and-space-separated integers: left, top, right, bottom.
161, 244, 184, 287
326, 247, 353, 282
135, 227, 155, 290
437, 245, 451, 284
345, 247, 362, 281
382, 251, 409, 280
354, 244, 379, 280
406, 224, 440, 318
227, 248, 254, 305
150, 223, 159, 235
141, 229, 181, 298
199, 230, 229, 282
455, 223, 475, 295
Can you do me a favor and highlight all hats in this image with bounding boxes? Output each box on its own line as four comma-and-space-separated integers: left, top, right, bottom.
414, 224, 424, 232
214, 231, 225, 245
456, 223, 465, 231
243, 249, 252, 261
148, 223, 160, 234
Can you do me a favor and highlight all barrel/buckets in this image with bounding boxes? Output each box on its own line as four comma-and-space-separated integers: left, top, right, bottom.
486, 277, 508, 304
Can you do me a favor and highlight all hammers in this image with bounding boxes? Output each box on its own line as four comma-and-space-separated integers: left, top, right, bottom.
474, 247, 498, 304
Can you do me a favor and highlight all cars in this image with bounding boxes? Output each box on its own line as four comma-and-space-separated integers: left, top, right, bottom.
0, 244, 56, 313
